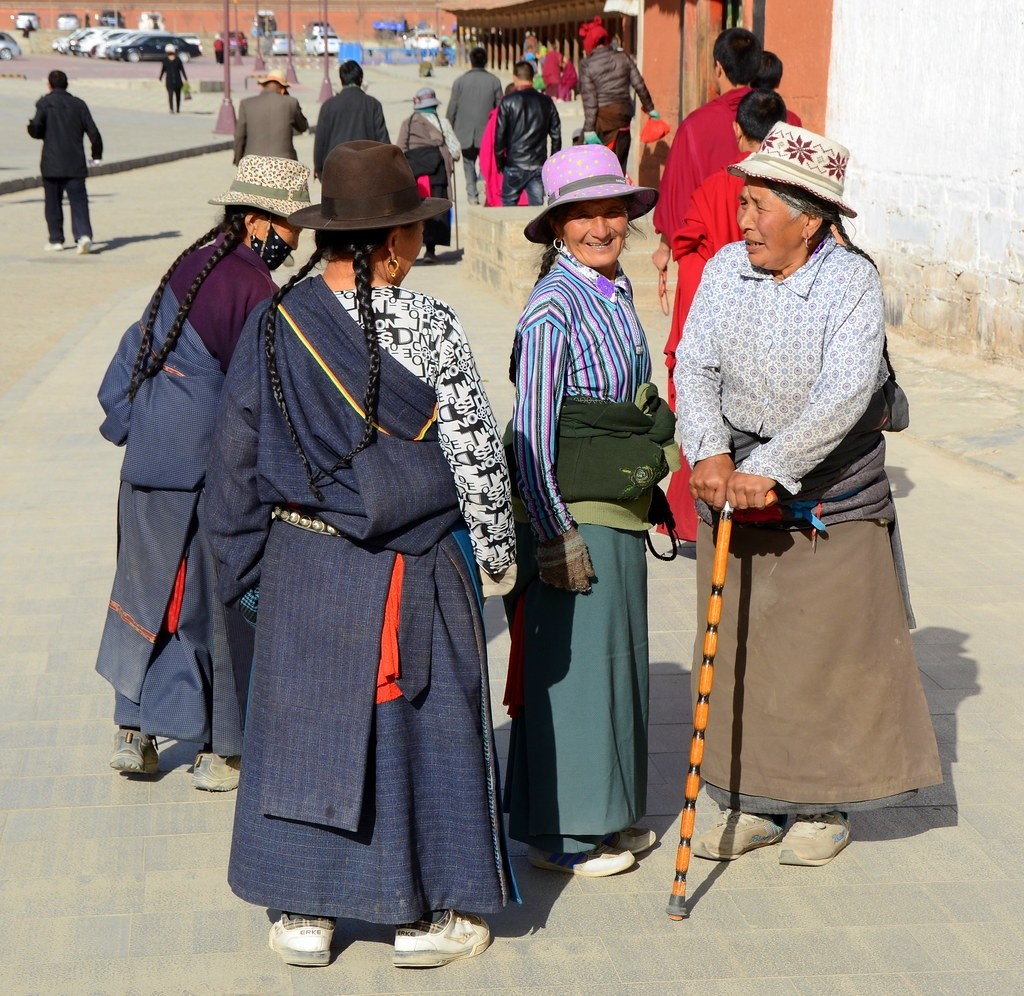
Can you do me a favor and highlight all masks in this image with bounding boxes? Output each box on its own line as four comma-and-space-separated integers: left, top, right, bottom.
251, 221, 292, 270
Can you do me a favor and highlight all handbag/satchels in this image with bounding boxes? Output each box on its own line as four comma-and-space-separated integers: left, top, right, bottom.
532, 74, 546, 90
184, 85, 189, 99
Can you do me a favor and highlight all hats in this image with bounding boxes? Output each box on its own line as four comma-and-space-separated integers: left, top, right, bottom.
287, 139, 453, 229
414, 88, 442, 110
207, 153, 313, 216
727, 120, 857, 219
524, 145, 659, 244
579, 16, 608, 53
258, 71, 289, 88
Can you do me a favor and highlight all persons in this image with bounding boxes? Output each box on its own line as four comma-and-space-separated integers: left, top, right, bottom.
192, 144, 519, 968
661, 116, 940, 868
28, 70, 107, 255
159, 43, 189, 114
94, 152, 315, 794
490, 140, 686, 878
213, 16, 799, 306
666, 91, 809, 529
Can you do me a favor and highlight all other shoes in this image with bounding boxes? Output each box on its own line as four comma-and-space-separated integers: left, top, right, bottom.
47, 243, 63, 249
77, 234, 91, 254
423, 251, 438, 263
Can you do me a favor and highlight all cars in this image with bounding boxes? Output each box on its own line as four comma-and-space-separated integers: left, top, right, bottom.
219, 31, 249, 56
401, 29, 441, 51
56, 14, 83, 31
269, 32, 298, 57
302, 20, 342, 57
0, 32, 22, 61
99, 10, 126, 28
137, 12, 166, 30
51, 26, 202, 64
15, 12, 41, 31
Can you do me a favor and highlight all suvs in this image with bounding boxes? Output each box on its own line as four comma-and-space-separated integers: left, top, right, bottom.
251, 10, 277, 35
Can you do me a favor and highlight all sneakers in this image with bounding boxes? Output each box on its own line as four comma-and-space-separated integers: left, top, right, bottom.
589, 826, 658, 854
391, 908, 490, 967
778, 812, 850, 866
191, 750, 243, 791
527, 840, 635, 876
267, 910, 335, 967
691, 808, 788, 860
109, 729, 159, 773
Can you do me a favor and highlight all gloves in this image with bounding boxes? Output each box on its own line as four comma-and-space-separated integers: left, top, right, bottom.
583, 132, 600, 144
537, 528, 596, 592
648, 110, 661, 120
